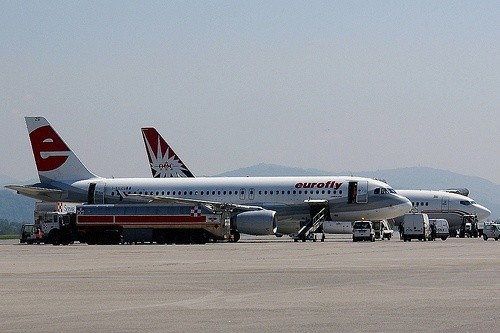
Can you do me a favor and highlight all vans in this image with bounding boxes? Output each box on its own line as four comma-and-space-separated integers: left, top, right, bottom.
429, 219, 450, 241
403, 213, 432, 242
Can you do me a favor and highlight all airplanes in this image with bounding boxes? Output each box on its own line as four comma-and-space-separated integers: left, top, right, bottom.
3, 117, 413, 237
323, 188, 492, 234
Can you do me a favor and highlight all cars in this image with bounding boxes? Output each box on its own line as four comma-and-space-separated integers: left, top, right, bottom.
483, 225, 500, 241
352, 220, 375, 242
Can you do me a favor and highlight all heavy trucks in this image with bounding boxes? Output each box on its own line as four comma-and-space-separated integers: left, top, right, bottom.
38, 202, 234, 246
